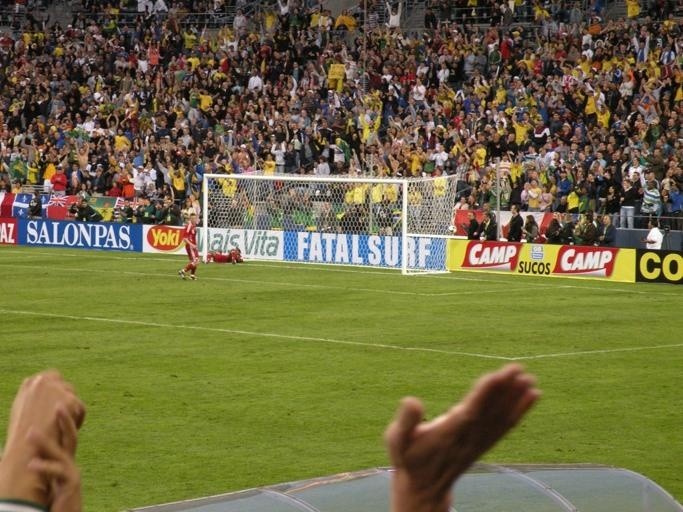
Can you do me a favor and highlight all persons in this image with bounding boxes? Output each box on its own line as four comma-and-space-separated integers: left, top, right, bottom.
0, 366, 88, 512
177, 213, 199, 279
382, 363, 544, 511
1, 1, 683, 248
201, 246, 243, 265
